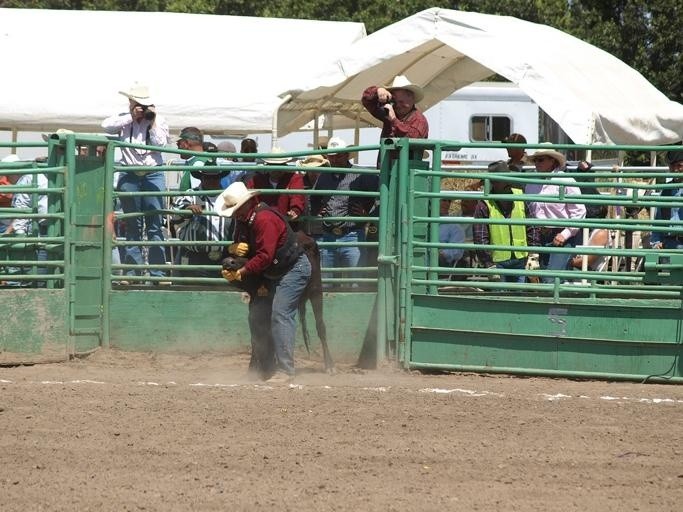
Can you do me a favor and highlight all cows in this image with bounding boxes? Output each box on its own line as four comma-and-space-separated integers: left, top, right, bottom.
221, 231, 336, 376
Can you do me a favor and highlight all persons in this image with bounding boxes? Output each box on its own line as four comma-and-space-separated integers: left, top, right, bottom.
360, 74, 430, 170
98, 81, 175, 286
210, 180, 312, 388
1, 126, 682, 289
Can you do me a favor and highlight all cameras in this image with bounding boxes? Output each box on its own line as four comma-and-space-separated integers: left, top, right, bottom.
377, 100, 397, 117
136, 105, 155, 121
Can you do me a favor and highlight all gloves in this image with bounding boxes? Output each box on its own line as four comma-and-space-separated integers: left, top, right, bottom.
222, 270, 241, 282
228, 243, 248, 257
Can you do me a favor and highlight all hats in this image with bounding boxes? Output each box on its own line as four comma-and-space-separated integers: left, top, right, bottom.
119, 84, 155, 106
527, 142, 565, 167
42, 129, 78, 155
214, 182, 260, 217
382, 75, 423, 103
260, 137, 357, 167
501, 134, 526, 143
191, 160, 230, 179
